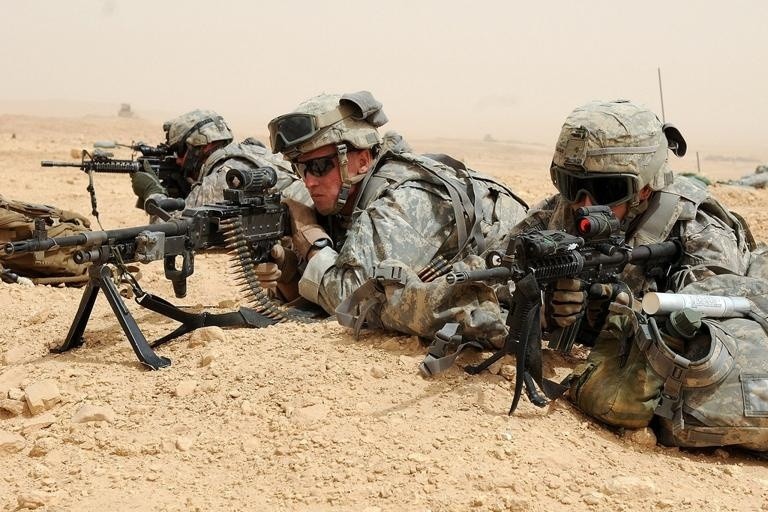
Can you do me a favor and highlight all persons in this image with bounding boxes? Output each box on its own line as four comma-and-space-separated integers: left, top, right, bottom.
477, 98, 768, 332
129, 109, 315, 224
253, 91, 530, 349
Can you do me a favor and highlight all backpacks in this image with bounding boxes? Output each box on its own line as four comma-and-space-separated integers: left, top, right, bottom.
658, 273, 767, 451
0, 197, 95, 285
333, 255, 508, 377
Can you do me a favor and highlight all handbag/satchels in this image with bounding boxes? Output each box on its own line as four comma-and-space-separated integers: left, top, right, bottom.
569, 294, 674, 429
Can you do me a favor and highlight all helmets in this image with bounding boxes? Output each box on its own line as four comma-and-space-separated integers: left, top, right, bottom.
163, 109, 233, 146
553, 98, 673, 192
278, 91, 383, 161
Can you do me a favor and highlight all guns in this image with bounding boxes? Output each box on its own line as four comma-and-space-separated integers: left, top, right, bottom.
41, 140, 191, 209
446, 205, 686, 407
5, 167, 293, 371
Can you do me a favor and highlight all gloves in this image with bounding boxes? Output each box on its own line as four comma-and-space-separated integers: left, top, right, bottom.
586, 282, 642, 339
130, 159, 169, 201
255, 244, 297, 288
280, 197, 334, 266
539, 277, 589, 331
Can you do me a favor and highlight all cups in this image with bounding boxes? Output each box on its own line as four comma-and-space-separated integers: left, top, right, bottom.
658, 308, 702, 344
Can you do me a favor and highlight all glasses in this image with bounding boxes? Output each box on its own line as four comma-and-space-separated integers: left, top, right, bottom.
550, 132, 670, 209
291, 148, 357, 179
177, 116, 223, 158
268, 104, 354, 153
